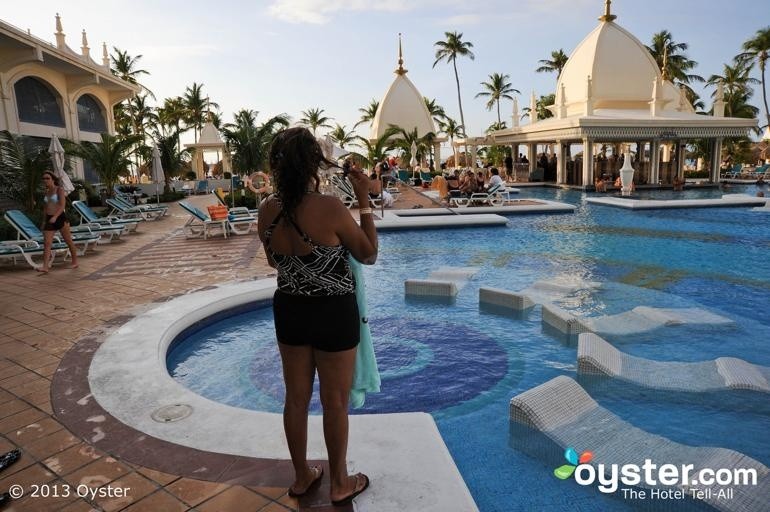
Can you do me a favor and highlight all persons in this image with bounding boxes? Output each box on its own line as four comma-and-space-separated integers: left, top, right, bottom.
670, 144, 676, 160
342, 150, 641, 203
257, 127, 379, 507
719, 161, 768, 197
36, 169, 80, 273
672, 176, 679, 191
206, 173, 212, 179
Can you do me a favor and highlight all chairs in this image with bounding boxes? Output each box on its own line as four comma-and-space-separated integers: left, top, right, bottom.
746, 164, 770, 179
405, 265, 770, 512
0, 195, 169, 270
721, 162, 743, 179
328, 167, 510, 210
179, 176, 259, 240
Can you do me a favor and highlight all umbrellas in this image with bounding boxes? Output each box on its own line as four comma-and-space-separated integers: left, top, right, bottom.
409, 139, 418, 182
47, 131, 76, 213
151, 143, 166, 207
323, 131, 335, 196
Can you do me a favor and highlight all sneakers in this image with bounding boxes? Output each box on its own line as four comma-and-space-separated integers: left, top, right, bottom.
0, 491, 13, 504
0, 450, 21, 469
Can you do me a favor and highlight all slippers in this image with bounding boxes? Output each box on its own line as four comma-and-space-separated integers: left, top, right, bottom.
331, 473, 369, 505
289, 465, 322, 496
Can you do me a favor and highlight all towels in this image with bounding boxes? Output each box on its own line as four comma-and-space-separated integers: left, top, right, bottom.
349, 251, 381, 410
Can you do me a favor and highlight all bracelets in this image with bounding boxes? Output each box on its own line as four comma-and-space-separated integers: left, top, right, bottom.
358, 207, 373, 215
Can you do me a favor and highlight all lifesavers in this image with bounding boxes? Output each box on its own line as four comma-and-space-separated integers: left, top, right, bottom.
248, 173, 270, 193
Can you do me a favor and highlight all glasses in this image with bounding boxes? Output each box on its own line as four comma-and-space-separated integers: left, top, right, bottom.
42, 177, 50, 180
